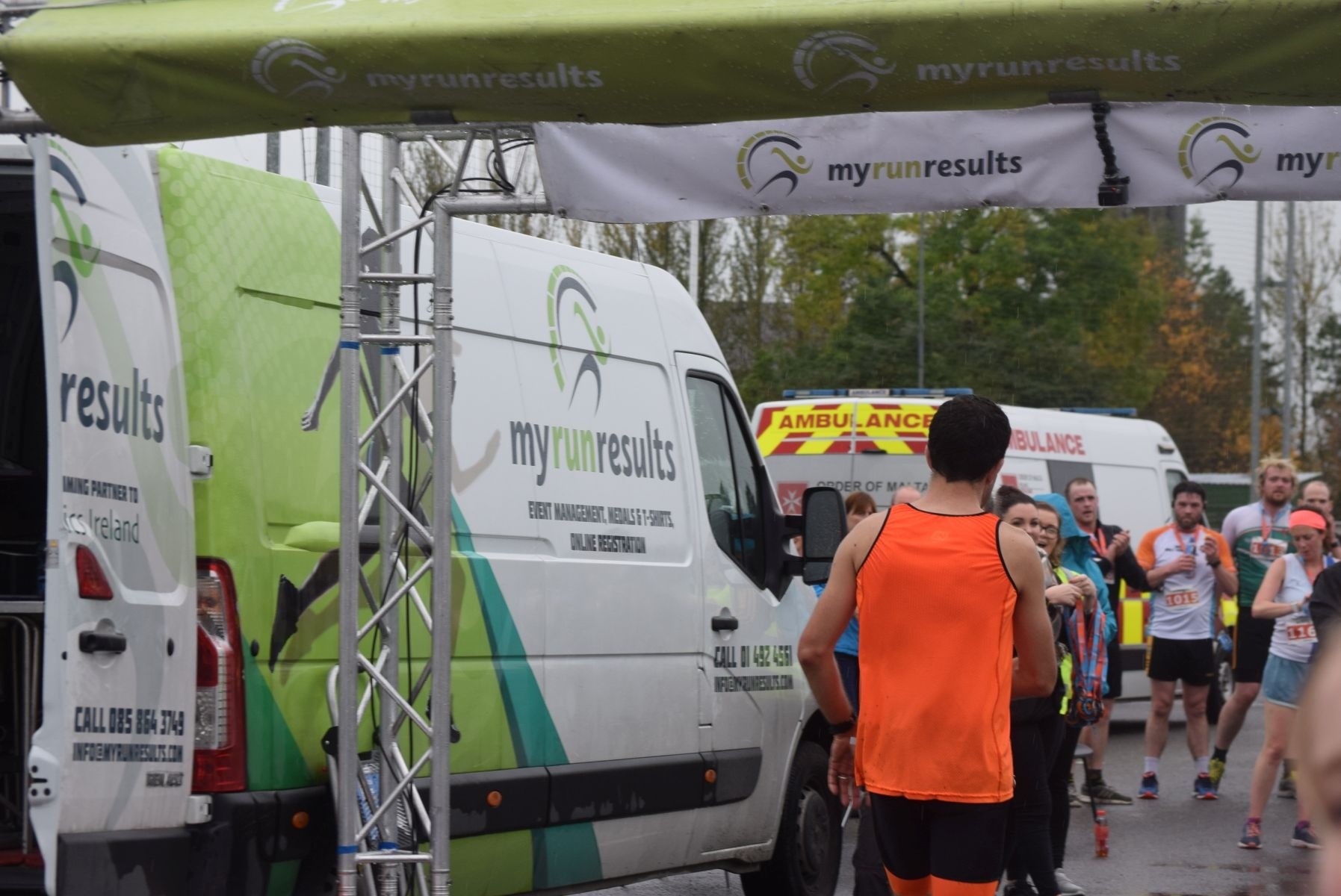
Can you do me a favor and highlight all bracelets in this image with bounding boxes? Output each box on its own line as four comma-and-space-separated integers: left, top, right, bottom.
1329, 542, 1339, 548
1207, 561, 1221, 567
829, 711, 858, 735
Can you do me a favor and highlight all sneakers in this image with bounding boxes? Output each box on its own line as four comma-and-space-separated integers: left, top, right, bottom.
1237, 822, 1263, 849
1191, 773, 1220, 799
1137, 771, 1159, 798
1290, 823, 1325, 850
1079, 783, 1133, 804
1069, 778, 1080, 806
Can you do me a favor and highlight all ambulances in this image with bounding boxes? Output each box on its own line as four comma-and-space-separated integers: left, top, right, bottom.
749, 385, 1240, 728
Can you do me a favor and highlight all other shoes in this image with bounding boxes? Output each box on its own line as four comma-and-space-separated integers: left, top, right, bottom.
1276, 769, 1297, 798
1208, 758, 1225, 787
1004, 881, 1038, 896
1055, 868, 1086, 896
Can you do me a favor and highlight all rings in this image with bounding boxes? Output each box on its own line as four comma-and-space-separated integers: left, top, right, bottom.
838, 776, 848, 779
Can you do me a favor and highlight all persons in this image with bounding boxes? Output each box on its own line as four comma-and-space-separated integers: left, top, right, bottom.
1290, 560, 1341, 896
983, 484, 1117, 896
797, 395, 1057, 895
1296, 479, 1341, 560
815, 487, 920, 818
1064, 478, 1145, 806
1238, 504, 1341, 849
1210, 459, 1296, 798
1138, 481, 1240, 798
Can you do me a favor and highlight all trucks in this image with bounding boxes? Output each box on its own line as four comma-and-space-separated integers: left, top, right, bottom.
0, 124, 858, 894
1188, 468, 1322, 540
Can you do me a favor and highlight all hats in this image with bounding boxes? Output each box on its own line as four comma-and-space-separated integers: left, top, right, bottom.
1032, 493, 1097, 565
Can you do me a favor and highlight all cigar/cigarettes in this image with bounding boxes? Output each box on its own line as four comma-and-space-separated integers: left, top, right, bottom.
841, 799, 853, 827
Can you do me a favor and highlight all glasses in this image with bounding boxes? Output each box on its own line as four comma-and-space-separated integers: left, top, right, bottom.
1040, 526, 1059, 539
